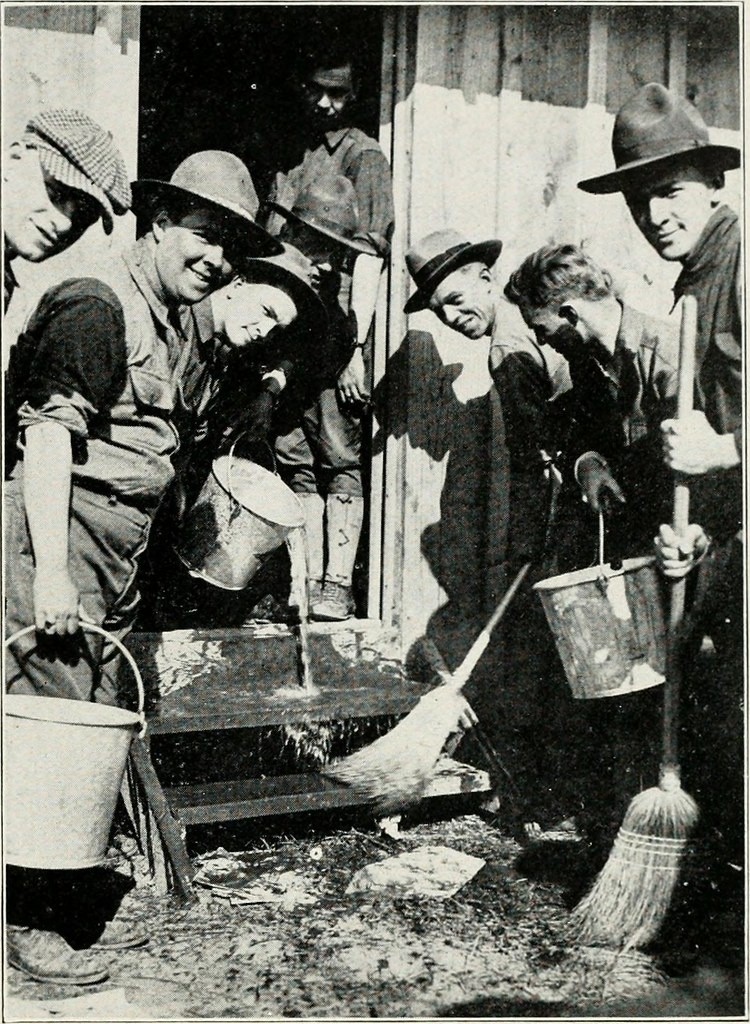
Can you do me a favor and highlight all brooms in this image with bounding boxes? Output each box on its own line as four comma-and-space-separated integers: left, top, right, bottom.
318, 563, 534, 821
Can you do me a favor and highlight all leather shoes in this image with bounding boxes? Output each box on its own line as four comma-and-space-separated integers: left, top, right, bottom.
6, 923, 111, 985
89, 920, 151, 950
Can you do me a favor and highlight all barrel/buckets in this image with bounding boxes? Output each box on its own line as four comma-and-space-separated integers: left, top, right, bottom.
2, 619, 146, 870
529, 505, 666, 700
172, 430, 307, 591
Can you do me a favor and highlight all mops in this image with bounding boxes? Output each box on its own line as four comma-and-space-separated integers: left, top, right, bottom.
550, 296, 701, 960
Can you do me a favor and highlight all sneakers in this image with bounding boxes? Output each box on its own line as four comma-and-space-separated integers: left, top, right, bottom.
305, 578, 357, 621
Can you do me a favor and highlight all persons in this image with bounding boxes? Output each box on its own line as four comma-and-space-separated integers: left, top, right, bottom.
5, 42, 391, 987
400, 88, 744, 979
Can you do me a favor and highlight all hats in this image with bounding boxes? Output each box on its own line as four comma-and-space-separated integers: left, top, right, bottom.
257, 172, 382, 258
19, 107, 134, 236
244, 240, 334, 346
400, 227, 504, 316
122, 146, 290, 263
576, 82, 741, 195
